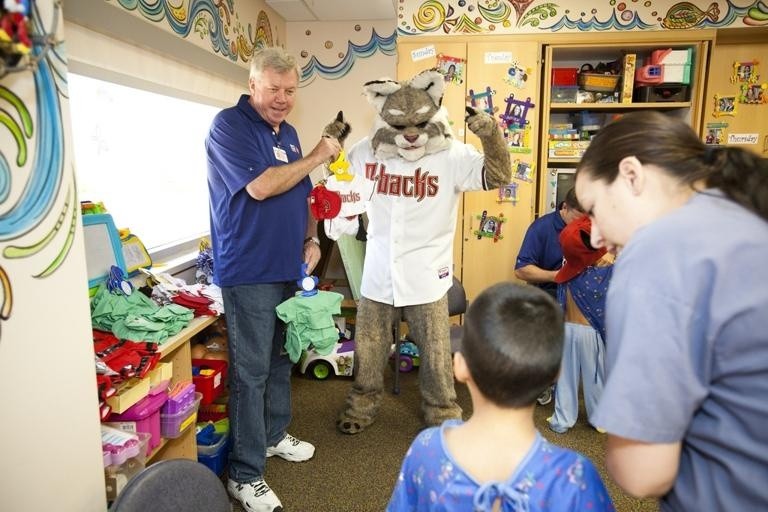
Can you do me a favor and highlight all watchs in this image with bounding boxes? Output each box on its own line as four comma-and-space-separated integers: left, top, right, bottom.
304, 236, 322, 247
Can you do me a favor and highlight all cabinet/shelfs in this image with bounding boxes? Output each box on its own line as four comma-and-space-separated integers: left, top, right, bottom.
97, 307, 230, 506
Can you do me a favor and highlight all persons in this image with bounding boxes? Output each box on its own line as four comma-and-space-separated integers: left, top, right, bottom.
203, 44, 343, 512
473, 68, 533, 243
574, 109, 768, 512
444, 65, 456, 80
515, 186, 587, 407
543, 215, 616, 435
704, 61, 763, 145
386, 280, 618, 512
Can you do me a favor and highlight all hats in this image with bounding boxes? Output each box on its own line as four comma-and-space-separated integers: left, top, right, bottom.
554, 216, 608, 284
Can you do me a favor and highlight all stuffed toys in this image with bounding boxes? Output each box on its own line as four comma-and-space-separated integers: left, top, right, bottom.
321, 68, 514, 434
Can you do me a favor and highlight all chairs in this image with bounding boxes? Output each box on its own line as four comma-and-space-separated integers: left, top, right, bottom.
108, 457, 231, 512
392, 274, 467, 394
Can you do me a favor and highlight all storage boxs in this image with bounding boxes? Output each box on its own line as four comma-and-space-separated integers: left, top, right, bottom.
105, 359, 231, 506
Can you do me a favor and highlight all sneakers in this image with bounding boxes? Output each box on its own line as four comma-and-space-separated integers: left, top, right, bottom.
227, 472, 284, 512
266, 433, 316, 462
